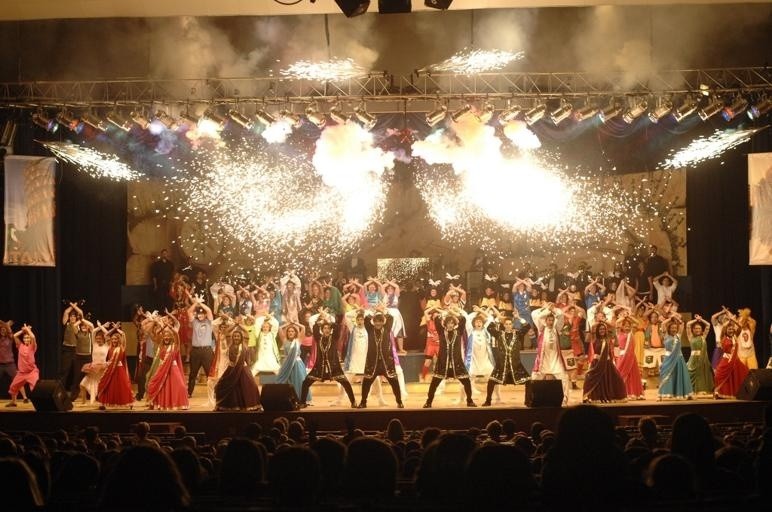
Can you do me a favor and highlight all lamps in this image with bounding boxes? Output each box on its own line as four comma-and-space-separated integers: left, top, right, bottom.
131, 107, 150, 131
281, 109, 305, 128
622, 101, 647, 122
549, 102, 574, 125
153, 112, 177, 132
648, 101, 674, 123
721, 98, 750, 121
451, 106, 475, 123
33, 112, 59, 133
203, 113, 224, 127
305, 107, 326, 129
745, 98, 771, 120
423, 107, 448, 127
573, 103, 600, 121
474, 103, 495, 122
78, 110, 108, 132
329, 106, 350, 126
108, 109, 133, 134
55, 112, 80, 131
255, 109, 277, 128
353, 107, 378, 130
671, 100, 699, 122
597, 101, 623, 122
522, 104, 547, 125
180, 116, 199, 131
497, 105, 522, 127
226, 108, 254, 131
696, 99, 725, 120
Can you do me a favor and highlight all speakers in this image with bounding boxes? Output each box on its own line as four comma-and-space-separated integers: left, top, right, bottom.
378, 0, 412, 15
335, 0, 370, 18
424, 0, 453, 11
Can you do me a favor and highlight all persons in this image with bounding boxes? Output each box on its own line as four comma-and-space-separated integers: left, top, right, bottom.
610, 261, 624, 278
642, 305, 665, 376
335, 308, 390, 407
608, 278, 638, 310
524, 277, 544, 309
72, 317, 94, 400
513, 275, 532, 331
207, 310, 236, 406
342, 292, 360, 314
96, 322, 135, 411
80, 321, 111, 406
555, 288, 574, 308
544, 263, 563, 303
420, 279, 442, 341
585, 299, 611, 326
253, 283, 271, 314
738, 309, 758, 369
322, 280, 342, 314
584, 319, 627, 404
0, 320, 30, 403
444, 273, 462, 290
423, 310, 478, 408
7, 324, 40, 406
135, 307, 157, 400
662, 299, 681, 318
281, 270, 302, 322
364, 276, 385, 307
531, 303, 570, 406
360, 305, 404, 408
188, 295, 216, 398
632, 302, 649, 375
307, 275, 324, 314
241, 314, 256, 365
300, 308, 358, 407
656, 314, 693, 401
455, 304, 504, 405
168, 278, 191, 363
146, 320, 190, 410
486, 307, 500, 317
625, 243, 641, 279
276, 321, 313, 406
644, 244, 664, 273
152, 248, 172, 309
715, 315, 750, 399
557, 314, 579, 391
562, 302, 584, 375
343, 277, 365, 298
614, 312, 646, 400
217, 293, 236, 314
565, 286, 581, 305
190, 271, 207, 303
60, 295, 87, 387
212, 322, 261, 412
420, 306, 441, 383
480, 274, 499, 306
252, 313, 284, 378
711, 305, 736, 374
584, 276, 605, 308
446, 285, 467, 311
484, 310, 531, 406
635, 261, 653, 302
212, 280, 233, 313
1, 410, 772, 512
653, 271, 678, 303
382, 278, 405, 352
165, 309, 182, 332
686, 314, 716, 398
238, 285, 254, 315
